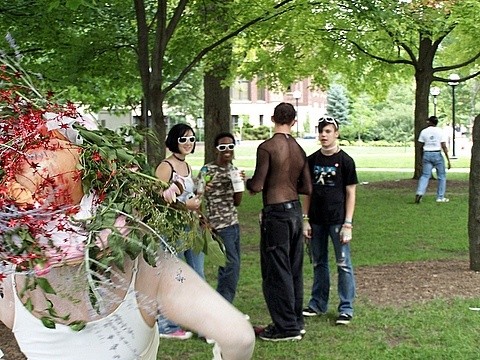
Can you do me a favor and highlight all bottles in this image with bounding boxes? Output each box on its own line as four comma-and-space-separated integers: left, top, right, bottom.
197, 166, 207, 198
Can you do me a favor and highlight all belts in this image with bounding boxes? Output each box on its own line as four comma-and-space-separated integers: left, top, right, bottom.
427, 150, 441, 152
263, 200, 302, 212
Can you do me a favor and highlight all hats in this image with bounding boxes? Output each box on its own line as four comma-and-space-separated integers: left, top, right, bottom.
427, 116, 438, 125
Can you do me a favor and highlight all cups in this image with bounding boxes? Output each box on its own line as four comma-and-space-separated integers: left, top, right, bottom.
227, 169, 246, 193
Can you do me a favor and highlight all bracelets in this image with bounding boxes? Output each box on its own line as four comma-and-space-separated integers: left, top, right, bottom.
302, 214, 309, 223
342, 224, 352, 228
344, 221, 352, 223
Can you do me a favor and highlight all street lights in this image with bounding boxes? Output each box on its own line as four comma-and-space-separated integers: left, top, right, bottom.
447, 73, 461, 156
293, 90, 302, 137
430, 85, 441, 116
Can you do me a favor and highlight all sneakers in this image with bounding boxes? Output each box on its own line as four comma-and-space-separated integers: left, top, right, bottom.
335, 312, 351, 324
302, 306, 317, 316
264, 324, 307, 334
158, 329, 194, 340
259, 325, 302, 341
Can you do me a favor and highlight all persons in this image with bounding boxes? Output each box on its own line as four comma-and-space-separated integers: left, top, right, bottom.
0, 51, 255, 360
415, 116, 451, 204
246, 102, 312, 341
154, 124, 214, 344
197, 133, 250, 321
302, 117, 359, 325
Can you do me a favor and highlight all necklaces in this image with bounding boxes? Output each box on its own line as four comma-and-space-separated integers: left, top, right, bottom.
173, 153, 185, 161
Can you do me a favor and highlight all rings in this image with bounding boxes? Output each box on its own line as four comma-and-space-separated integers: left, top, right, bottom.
243, 172, 245, 175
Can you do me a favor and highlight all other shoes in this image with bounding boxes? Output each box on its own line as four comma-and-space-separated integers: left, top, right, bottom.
415, 194, 421, 204
436, 197, 450, 202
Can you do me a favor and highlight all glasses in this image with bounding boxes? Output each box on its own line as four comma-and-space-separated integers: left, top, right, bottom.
216, 144, 235, 152
318, 117, 338, 129
177, 135, 196, 145
